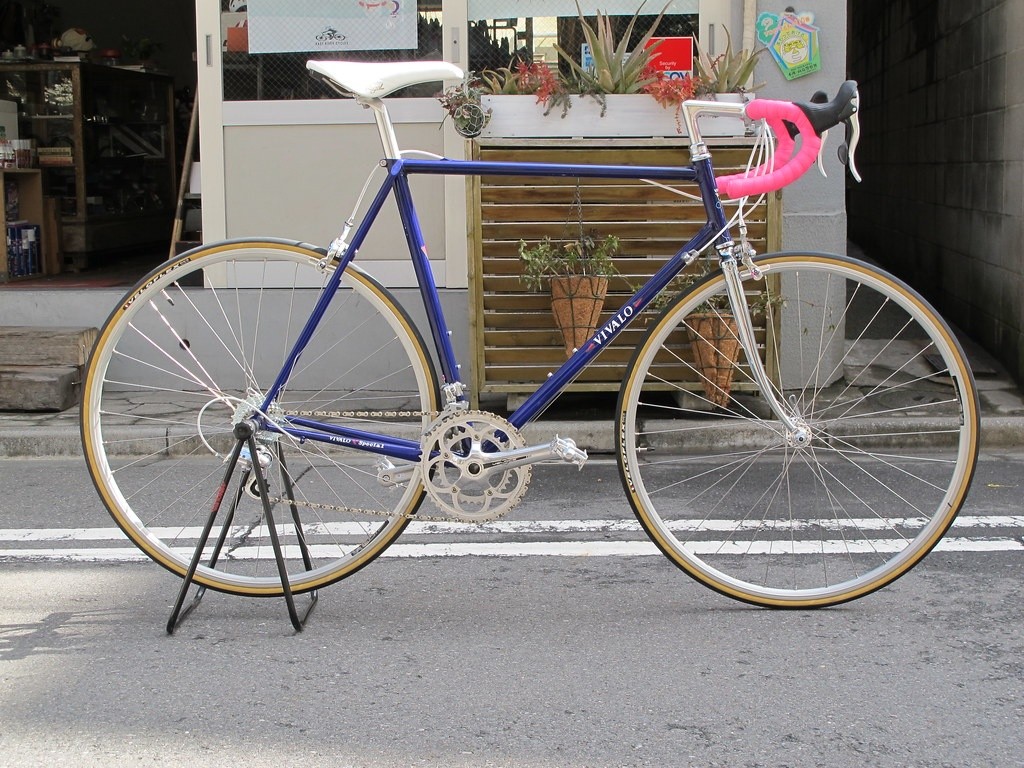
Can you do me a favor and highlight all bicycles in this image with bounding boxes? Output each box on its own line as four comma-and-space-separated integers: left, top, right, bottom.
79, 57, 984, 636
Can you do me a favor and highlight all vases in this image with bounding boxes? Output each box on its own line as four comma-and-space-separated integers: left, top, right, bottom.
479, 93, 756, 139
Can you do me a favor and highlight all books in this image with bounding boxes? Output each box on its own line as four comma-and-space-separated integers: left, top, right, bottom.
5, 140, 37, 169
7, 226, 40, 278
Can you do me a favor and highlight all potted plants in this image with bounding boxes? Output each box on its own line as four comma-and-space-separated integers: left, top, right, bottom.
514, 234, 641, 359
645, 244, 835, 413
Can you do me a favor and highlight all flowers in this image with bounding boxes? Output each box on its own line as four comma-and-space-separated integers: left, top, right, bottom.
438, 0, 771, 138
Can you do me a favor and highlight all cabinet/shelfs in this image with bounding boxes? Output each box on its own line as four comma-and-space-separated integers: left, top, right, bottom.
0, 168, 49, 282
167, 51, 303, 261
0, 58, 195, 274
